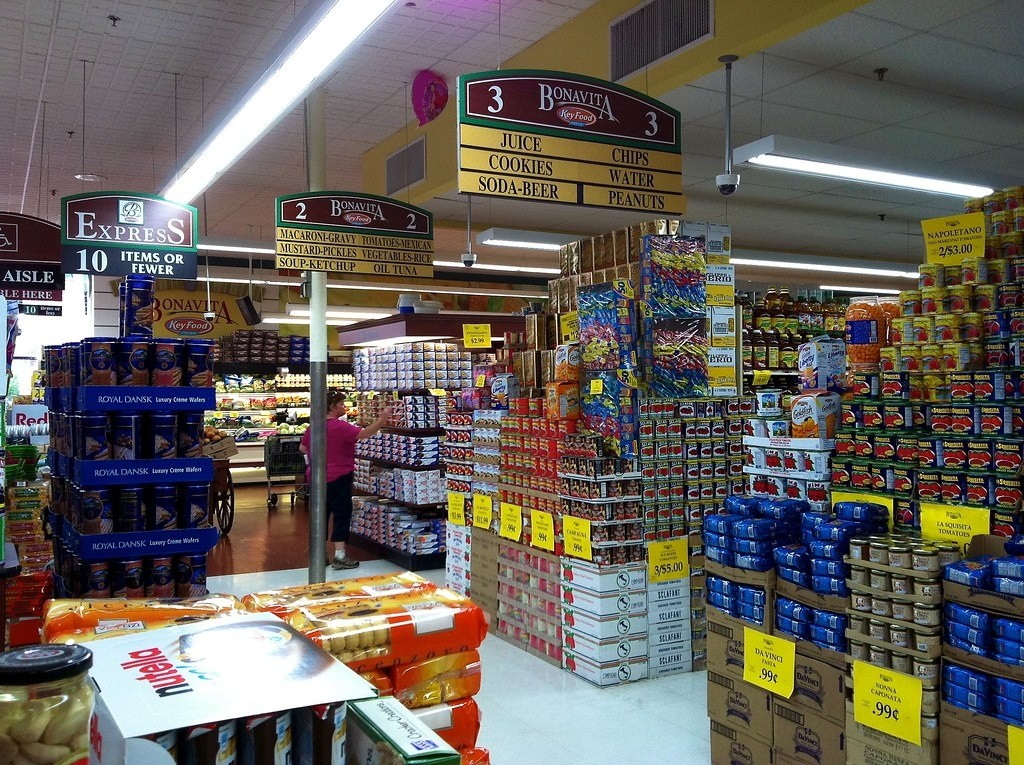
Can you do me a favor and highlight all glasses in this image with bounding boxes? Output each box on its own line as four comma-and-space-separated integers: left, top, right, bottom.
330, 390, 341, 404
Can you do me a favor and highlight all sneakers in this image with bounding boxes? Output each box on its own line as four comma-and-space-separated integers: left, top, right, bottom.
331, 554, 360, 570
324, 552, 330, 567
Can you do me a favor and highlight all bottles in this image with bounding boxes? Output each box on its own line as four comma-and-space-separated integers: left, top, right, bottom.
741, 282, 849, 393
5, 423, 49, 445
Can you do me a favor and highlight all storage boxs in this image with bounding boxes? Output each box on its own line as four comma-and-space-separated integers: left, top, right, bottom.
445, 218, 1024, 765
67, 611, 463, 765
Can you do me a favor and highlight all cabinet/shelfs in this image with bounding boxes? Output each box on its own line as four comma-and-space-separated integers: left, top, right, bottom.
0, 296, 499, 597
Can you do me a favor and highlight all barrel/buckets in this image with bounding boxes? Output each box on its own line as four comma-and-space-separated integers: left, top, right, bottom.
397, 293, 422, 314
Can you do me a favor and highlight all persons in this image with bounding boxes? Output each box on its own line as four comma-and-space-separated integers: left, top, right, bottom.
299, 391, 392, 570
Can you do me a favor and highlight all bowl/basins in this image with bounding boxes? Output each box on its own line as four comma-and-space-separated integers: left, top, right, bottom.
414, 300, 441, 314
529, 302, 544, 312
521, 306, 530, 316
